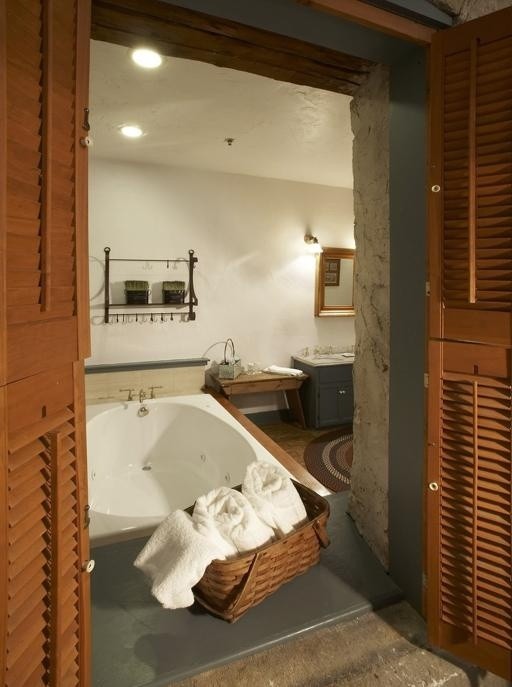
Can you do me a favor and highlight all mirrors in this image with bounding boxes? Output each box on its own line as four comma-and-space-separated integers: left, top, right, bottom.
315, 246, 355, 316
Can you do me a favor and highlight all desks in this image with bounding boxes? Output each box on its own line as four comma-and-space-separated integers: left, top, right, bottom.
209, 370, 309, 430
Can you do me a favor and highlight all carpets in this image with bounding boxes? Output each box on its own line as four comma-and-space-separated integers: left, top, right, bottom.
304, 427, 352, 492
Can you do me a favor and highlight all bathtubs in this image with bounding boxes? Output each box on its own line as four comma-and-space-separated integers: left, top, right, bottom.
86, 393, 299, 550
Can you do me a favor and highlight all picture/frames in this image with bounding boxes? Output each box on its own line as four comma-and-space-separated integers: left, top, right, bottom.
324, 258, 340, 286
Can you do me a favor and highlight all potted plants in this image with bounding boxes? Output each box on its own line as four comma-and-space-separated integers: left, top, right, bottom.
201, 338, 242, 379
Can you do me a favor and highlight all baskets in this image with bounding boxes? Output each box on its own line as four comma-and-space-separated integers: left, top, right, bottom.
184, 474, 330, 624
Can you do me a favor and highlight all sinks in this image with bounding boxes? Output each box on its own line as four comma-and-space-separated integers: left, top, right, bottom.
304, 354, 344, 361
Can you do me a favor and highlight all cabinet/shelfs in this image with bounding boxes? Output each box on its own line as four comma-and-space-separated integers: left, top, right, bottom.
294, 361, 353, 430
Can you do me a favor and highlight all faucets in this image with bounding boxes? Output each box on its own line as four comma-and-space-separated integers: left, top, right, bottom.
313, 345, 322, 355
138, 389, 147, 403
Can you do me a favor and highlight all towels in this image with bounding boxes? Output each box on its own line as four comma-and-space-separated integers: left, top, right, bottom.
133, 458, 310, 613
262, 364, 304, 378
